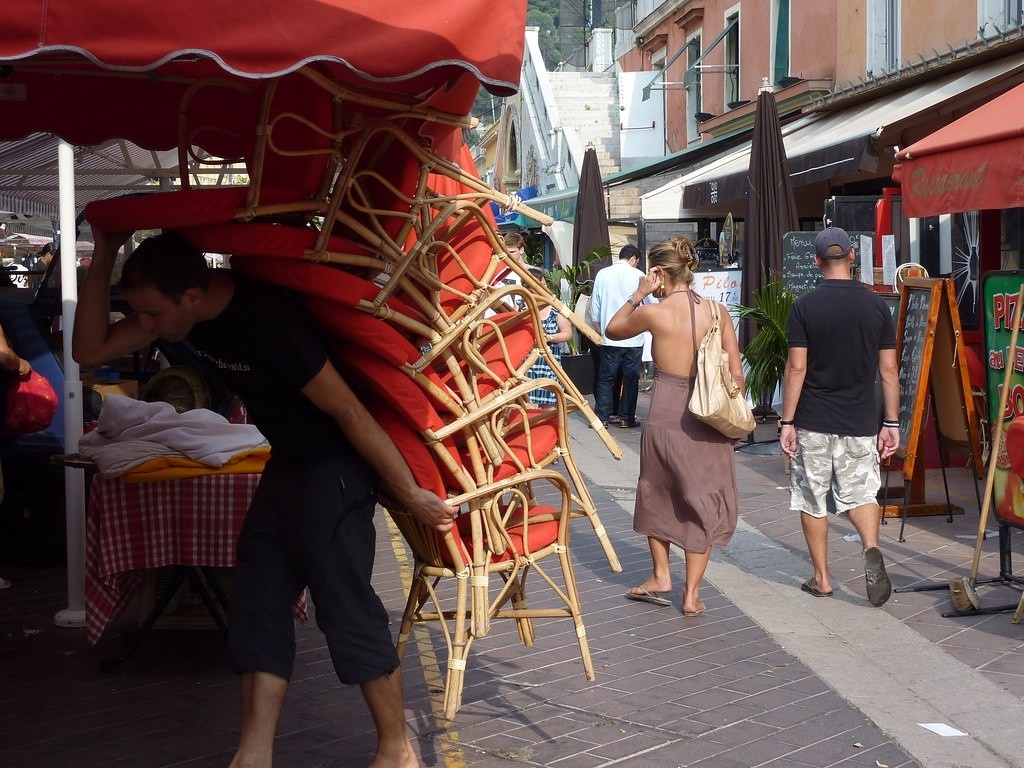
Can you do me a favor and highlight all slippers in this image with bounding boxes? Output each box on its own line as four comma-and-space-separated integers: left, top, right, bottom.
685, 600, 705, 616
863, 547, 891, 606
801, 577, 833, 597
625, 585, 672, 606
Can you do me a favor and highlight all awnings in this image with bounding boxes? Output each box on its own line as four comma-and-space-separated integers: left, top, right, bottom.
683, 49, 1024, 210
892, 81, 1024, 219
522, 110, 799, 227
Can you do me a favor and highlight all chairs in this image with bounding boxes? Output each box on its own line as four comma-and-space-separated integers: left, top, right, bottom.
83, 65, 626, 719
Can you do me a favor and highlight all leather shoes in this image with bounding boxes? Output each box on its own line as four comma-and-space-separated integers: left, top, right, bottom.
619, 419, 640, 428
589, 422, 607, 428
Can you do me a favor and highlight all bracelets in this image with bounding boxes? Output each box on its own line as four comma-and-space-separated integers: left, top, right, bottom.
882, 419, 899, 428
780, 419, 794, 425
627, 295, 639, 308
550, 334, 553, 342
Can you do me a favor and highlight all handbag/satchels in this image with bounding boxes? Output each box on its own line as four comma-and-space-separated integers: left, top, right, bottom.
688, 299, 756, 439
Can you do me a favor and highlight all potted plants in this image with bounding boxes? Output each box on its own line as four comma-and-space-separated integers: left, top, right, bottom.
543, 239, 621, 396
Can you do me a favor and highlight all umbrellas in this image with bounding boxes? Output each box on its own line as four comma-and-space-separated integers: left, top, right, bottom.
0, 0, 527, 624
572, 143, 613, 301
737, 78, 796, 357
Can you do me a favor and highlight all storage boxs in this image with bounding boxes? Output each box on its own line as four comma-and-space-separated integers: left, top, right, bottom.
82, 376, 140, 417
136, 566, 228, 630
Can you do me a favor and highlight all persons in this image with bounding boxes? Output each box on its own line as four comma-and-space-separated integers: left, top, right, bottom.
779, 227, 900, 605
70, 222, 459, 768
604, 233, 746, 615
0, 242, 170, 590
484, 231, 660, 428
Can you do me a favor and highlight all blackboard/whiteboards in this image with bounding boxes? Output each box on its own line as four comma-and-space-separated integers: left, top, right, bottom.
885, 277, 984, 480
781, 231, 878, 297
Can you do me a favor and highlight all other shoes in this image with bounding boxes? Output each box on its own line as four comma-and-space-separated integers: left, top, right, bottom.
610, 416, 619, 422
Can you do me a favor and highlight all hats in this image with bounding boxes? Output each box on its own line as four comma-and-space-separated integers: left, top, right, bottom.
815, 226, 850, 259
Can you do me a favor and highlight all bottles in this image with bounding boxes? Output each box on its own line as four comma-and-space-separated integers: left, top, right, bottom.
227, 395, 247, 425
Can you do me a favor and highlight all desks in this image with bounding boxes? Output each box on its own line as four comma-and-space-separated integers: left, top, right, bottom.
82, 469, 308, 674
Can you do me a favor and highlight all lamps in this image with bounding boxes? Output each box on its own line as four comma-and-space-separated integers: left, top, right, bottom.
778, 77, 805, 87
726, 100, 750, 109
694, 112, 716, 122
